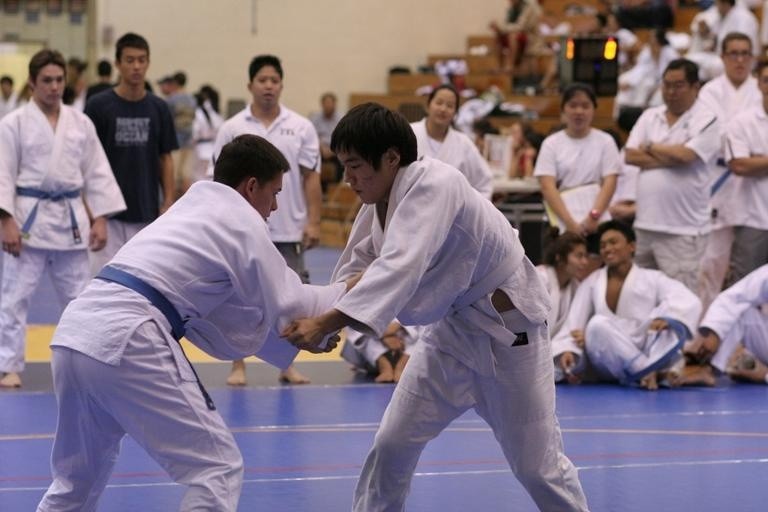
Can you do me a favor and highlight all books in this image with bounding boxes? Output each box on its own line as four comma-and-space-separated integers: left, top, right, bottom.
541, 183, 613, 235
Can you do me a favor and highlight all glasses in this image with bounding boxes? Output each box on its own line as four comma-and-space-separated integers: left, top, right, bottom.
724, 50, 751, 59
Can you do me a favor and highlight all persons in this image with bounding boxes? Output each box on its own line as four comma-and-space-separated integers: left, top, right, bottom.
697, 32, 759, 318
156, 77, 173, 95
408, 84, 493, 203
36, 138, 367, 511
488, 0, 546, 75
204, 53, 323, 387
276, 101, 590, 512
528, 231, 590, 386
531, 82, 622, 240
611, 108, 646, 220
722, 56, 768, 291
685, 0, 760, 80
671, 262, 768, 390
86, 60, 117, 99
163, 73, 195, 199
341, 315, 423, 386
83, 32, 181, 278
549, 219, 703, 391
541, 10, 680, 116
62, 55, 86, 110
506, 119, 541, 181
1, 77, 17, 117
306, 94, 350, 145
16, 81, 33, 109
0, 47, 128, 390
622, 58, 722, 292
472, 118, 507, 173
192, 85, 224, 179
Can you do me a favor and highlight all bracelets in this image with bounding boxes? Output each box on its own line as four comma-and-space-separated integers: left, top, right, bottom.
589, 208, 601, 220
